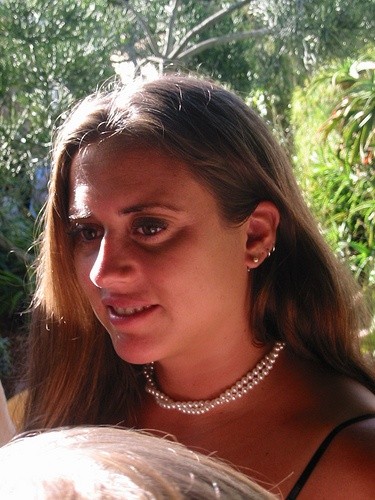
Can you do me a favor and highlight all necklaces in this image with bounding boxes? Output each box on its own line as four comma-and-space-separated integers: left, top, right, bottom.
143, 338, 286, 414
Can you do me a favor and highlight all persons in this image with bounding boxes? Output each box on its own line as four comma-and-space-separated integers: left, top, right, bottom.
0, 66, 375, 500
